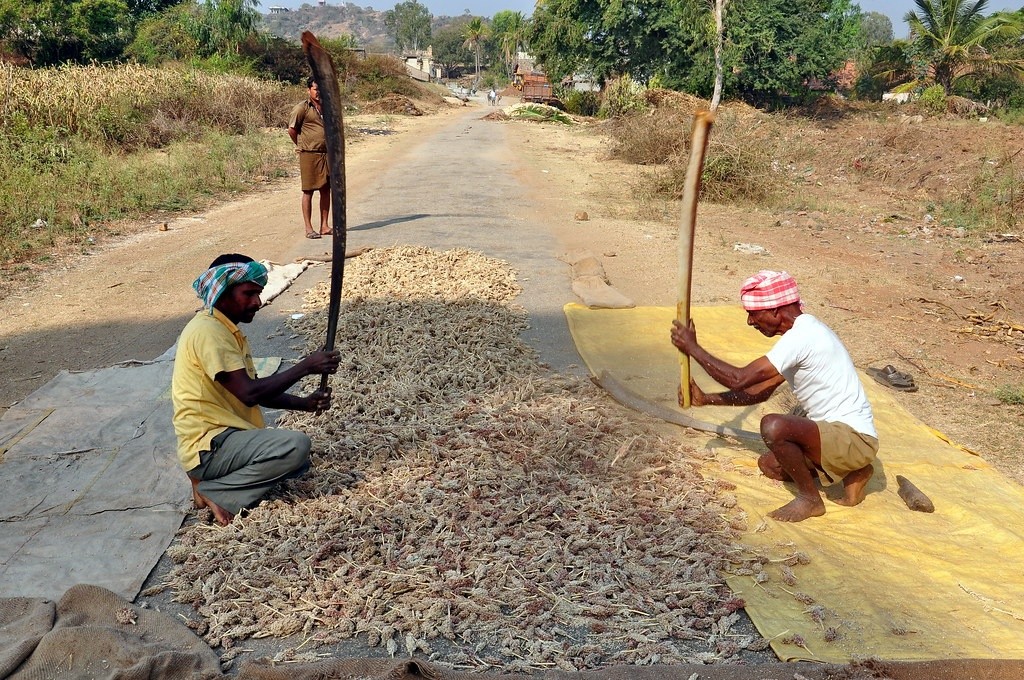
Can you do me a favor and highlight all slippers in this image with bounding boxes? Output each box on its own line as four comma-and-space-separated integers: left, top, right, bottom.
305, 231, 320, 238
896, 474, 934, 513
320, 228, 333, 234
866, 365, 919, 391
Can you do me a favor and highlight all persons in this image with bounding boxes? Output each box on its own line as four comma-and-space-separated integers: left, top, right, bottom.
172, 254, 341, 528
671, 270, 879, 523
288, 77, 335, 239
488, 89, 501, 106
